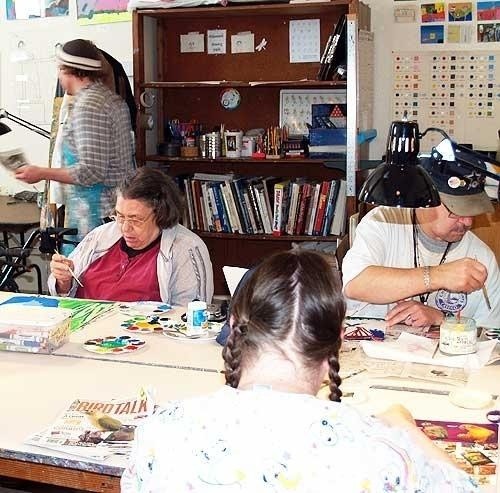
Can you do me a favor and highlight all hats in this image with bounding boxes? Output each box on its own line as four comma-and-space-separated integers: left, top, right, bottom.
420, 151, 495, 217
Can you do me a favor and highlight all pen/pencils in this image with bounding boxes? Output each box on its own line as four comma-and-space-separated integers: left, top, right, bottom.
482, 284, 491, 311
267, 125, 289, 155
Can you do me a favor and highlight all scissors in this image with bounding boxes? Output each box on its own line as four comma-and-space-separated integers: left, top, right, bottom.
168, 119, 207, 147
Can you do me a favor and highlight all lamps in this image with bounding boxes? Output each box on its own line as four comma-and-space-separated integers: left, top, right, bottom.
358, 119, 499, 208
0, 108, 54, 140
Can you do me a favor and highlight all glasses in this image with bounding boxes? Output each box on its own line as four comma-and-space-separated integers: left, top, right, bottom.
444, 205, 463, 220
109, 207, 155, 226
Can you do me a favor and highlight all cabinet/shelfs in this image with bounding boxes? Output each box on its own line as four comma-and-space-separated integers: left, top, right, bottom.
132, 0, 373, 302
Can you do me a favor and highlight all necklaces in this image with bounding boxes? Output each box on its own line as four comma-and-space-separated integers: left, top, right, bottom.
409, 209, 453, 305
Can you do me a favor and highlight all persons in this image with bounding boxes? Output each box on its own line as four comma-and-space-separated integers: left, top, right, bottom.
48, 168, 215, 307
120, 248, 477, 490
14, 38, 136, 258
341, 158, 499, 331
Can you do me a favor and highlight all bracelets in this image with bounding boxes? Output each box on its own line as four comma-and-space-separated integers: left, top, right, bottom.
421, 266, 432, 290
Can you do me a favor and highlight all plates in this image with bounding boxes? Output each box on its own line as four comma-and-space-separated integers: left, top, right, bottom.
163, 322, 225, 341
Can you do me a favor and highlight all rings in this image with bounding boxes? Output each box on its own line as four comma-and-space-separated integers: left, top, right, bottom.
406, 315, 410, 322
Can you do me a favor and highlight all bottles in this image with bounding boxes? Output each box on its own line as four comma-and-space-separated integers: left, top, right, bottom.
439, 316, 477, 356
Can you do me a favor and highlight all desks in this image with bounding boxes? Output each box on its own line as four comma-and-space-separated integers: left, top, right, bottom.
0, 292, 500, 492
0, 193, 66, 277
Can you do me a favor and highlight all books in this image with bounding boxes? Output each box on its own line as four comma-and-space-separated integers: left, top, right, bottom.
179, 173, 347, 236
394, 332, 438, 359
318, 13, 346, 80
23, 387, 160, 463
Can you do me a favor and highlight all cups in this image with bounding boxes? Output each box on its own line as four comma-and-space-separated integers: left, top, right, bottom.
186, 301, 208, 335
37, 192, 44, 209
224, 132, 243, 157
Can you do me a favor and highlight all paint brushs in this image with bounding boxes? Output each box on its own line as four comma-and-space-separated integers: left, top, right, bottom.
432, 343, 439, 358
53, 248, 84, 288
